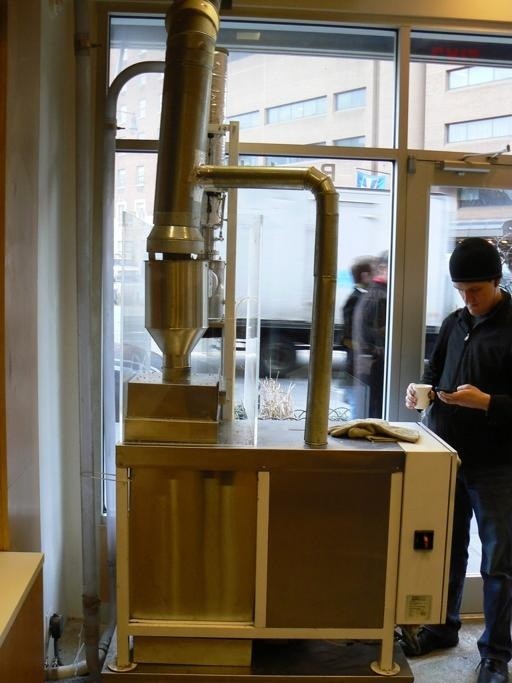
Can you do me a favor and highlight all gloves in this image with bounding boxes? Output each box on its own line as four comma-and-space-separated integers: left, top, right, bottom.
327, 417, 420, 444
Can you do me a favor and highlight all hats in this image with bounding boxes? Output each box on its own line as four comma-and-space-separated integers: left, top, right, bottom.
449, 237, 503, 284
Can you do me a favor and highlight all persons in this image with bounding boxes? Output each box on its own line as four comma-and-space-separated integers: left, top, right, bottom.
340, 251, 389, 419
398, 238, 512, 683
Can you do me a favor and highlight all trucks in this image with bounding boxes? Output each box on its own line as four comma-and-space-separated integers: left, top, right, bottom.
200, 186, 512, 380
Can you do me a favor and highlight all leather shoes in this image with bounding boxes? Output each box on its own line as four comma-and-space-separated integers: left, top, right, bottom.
475, 656, 509, 683
398, 616, 461, 658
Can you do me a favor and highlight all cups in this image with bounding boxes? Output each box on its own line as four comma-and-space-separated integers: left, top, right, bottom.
409, 382, 432, 409
